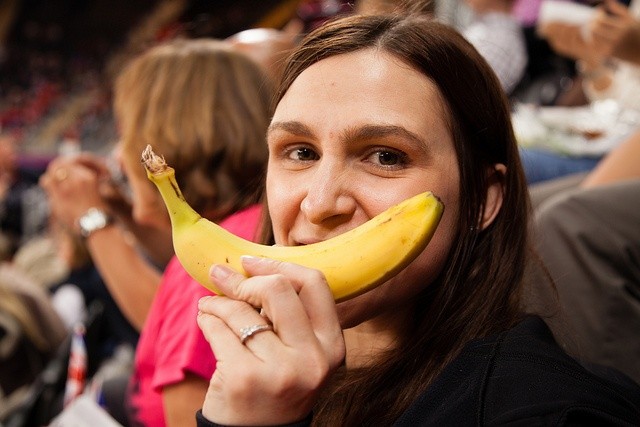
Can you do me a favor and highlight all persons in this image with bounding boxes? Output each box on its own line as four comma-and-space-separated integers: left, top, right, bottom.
196, 12, 615, 425
436, 0, 530, 94
38, 26, 290, 338
512, 1, 639, 385
512, 19, 639, 186
112, 40, 276, 427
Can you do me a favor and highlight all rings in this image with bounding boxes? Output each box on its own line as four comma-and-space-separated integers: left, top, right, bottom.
239, 320, 273, 346
53, 166, 68, 183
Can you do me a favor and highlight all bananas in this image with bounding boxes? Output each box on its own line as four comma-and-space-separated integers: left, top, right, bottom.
140, 144, 444, 307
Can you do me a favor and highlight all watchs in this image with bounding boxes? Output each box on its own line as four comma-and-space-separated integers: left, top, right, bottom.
78, 203, 116, 238
